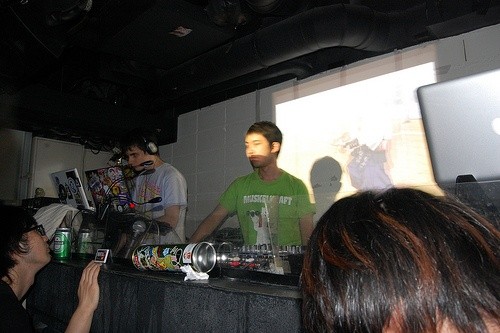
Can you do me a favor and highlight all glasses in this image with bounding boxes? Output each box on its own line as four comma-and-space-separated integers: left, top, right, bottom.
26, 223, 46, 236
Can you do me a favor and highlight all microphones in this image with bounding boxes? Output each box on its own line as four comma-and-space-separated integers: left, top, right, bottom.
120, 221, 146, 261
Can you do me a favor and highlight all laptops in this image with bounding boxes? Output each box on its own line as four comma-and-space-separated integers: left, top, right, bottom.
85, 165, 134, 214
418, 68, 500, 225
50, 168, 97, 212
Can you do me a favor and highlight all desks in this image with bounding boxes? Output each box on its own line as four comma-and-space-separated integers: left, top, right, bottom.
25, 252, 302, 333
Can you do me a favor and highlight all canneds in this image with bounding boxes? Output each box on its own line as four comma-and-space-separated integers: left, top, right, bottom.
53, 227, 71, 260
76, 228, 91, 253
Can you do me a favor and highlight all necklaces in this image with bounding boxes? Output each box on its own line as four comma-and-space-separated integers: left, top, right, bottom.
6, 281, 20, 300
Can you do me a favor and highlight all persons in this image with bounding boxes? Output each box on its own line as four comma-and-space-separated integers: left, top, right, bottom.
300, 187, 500, 333
190, 121, 315, 248
0, 204, 102, 333
114, 129, 187, 254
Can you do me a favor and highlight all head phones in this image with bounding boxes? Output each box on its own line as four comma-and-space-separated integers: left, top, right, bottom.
140, 137, 158, 153
